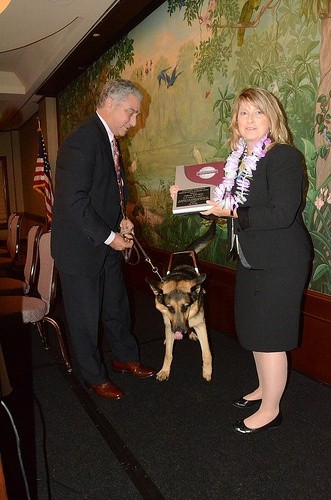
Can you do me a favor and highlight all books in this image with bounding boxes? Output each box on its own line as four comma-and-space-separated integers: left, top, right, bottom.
172, 160, 225, 215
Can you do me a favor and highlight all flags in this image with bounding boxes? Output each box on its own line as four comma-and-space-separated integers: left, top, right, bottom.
33, 130, 53, 229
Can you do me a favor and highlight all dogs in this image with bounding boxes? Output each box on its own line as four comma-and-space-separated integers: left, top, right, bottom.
142, 214, 219, 382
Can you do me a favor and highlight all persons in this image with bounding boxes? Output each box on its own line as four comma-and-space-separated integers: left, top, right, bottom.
169, 87, 311, 435
50, 79, 154, 398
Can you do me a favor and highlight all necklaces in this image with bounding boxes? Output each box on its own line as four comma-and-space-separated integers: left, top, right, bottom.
213, 134, 271, 210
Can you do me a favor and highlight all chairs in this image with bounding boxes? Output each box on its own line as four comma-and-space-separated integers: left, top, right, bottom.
0, 212, 73, 375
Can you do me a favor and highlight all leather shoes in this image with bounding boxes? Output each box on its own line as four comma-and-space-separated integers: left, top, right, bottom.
232, 395, 262, 408
111, 358, 156, 379
85, 379, 123, 398
231, 412, 281, 435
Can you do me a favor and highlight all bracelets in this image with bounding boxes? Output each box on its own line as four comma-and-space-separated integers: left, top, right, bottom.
230, 206, 235, 217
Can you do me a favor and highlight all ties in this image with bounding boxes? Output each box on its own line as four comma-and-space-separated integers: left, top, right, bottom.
111, 136, 125, 201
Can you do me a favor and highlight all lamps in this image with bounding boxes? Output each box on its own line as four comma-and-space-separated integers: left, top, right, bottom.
0, 71, 25, 95
